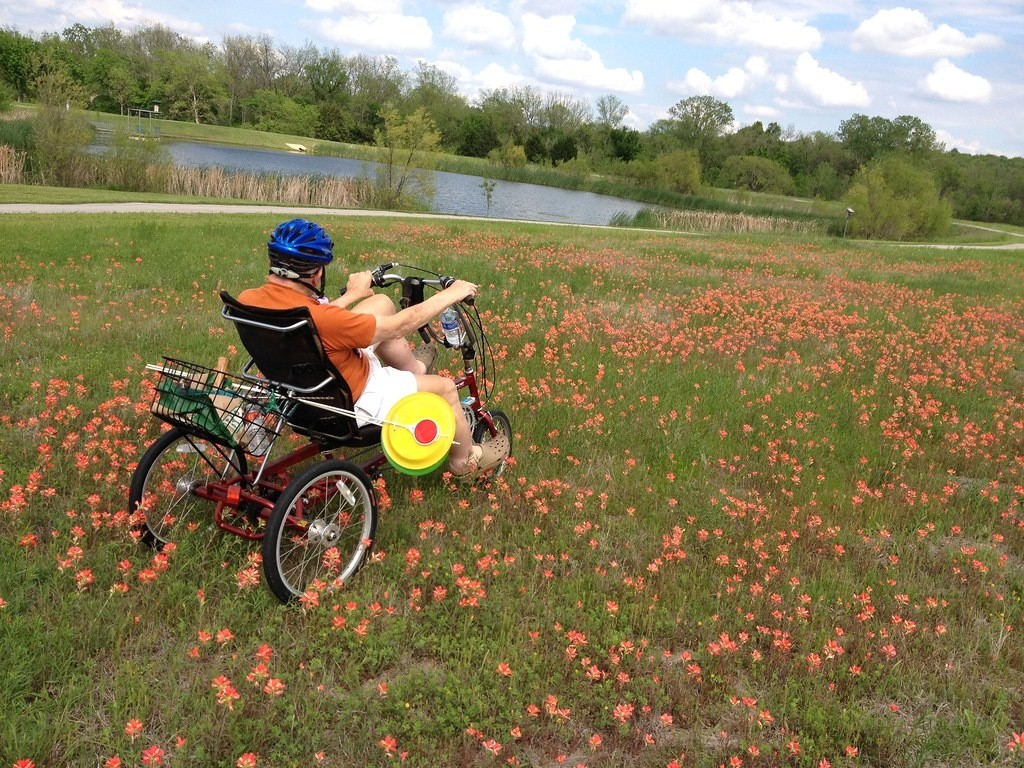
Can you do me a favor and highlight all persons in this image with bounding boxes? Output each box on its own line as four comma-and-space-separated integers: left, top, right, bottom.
234, 218, 511, 483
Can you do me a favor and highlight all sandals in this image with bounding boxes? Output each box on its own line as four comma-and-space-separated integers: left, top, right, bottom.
413, 343, 438, 374
448, 435, 510, 481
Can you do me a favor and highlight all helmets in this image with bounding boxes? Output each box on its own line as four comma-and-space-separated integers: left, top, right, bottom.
267, 218, 334, 278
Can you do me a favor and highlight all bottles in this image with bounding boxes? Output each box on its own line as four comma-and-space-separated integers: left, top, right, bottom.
242, 404, 270, 456
440, 308, 464, 347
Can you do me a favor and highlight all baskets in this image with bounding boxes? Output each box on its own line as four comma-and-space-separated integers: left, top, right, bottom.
151, 355, 301, 457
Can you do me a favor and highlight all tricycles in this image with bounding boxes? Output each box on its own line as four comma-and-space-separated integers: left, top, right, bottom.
128, 260, 513, 611
